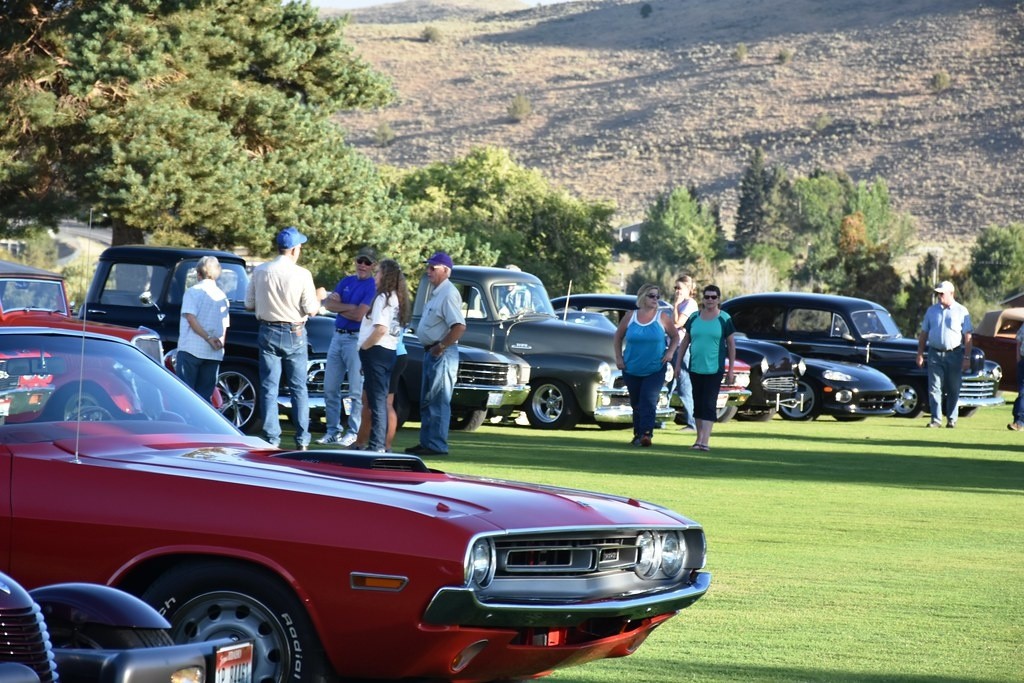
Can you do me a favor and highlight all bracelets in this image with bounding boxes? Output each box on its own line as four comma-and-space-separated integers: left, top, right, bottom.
205, 335, 212, 340
964, 356, 970, 359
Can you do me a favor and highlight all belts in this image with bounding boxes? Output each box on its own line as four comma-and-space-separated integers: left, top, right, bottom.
336, 327, 360, 333
423, 343, 438, 351
260, 320, 305, 326
931, 345, 961, 352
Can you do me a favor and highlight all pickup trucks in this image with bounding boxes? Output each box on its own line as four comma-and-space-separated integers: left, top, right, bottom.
408, 265, 676, 432
79, 244, 363, 436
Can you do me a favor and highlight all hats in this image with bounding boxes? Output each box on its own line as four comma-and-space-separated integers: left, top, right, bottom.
356, 247, 376, 263
423, 252, 453, 269
933, 280, 954, 294
275, 226, 307, 250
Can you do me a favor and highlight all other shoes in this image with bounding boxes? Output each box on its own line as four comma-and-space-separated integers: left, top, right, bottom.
946, 421, 954, 428
926, 421, 942, 428
413, 448, 448, 456
404, 444, 425, 454
676, 426, 695, 431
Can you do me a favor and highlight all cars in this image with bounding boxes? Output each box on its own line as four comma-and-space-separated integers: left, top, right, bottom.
0, 569, 254, 683
0, 327, 712, 683
536, 293, 807, 424
670, 356, 752, 407
718, 292, 1006, 419
392, 331, 530, 432
776, 355, 899, 422
0, 260, 224, 424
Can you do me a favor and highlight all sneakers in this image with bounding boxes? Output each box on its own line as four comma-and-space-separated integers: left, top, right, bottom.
1007, 422, 1024, 431
313, 431, 343, 444
641, 432, 654, 446
626, 433, 642, 448
333, 432, 358, 446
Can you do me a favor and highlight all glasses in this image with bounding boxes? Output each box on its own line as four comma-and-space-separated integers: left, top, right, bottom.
704, 295, 718, 299
356, 258, 372, 266
673, 286, 682, 290
427, 266, 445, 271
645, 293, 660, 299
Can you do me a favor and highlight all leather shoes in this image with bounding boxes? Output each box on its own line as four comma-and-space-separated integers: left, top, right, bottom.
298, 445, 309, 451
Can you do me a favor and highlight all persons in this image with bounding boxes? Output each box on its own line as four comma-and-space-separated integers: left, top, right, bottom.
916, 280, 974, 428
488, 264, 536, 425
315, 247, 377, 446
675, 286, 735, 451
405, 250, 466, 456
613, 282, 679, 446
1007, 322, 1024, 431
344, 259, 407, 455
826, 315, 844, 333
654, 276, 699, 431
176, 256, 230, 426
244, 227, 327, 451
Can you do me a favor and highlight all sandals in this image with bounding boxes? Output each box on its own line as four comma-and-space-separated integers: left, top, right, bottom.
691, 443, 701, 450
701, 443, 710, 451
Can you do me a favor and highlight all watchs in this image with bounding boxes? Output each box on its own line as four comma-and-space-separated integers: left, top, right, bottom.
439, 342, 447, 350
